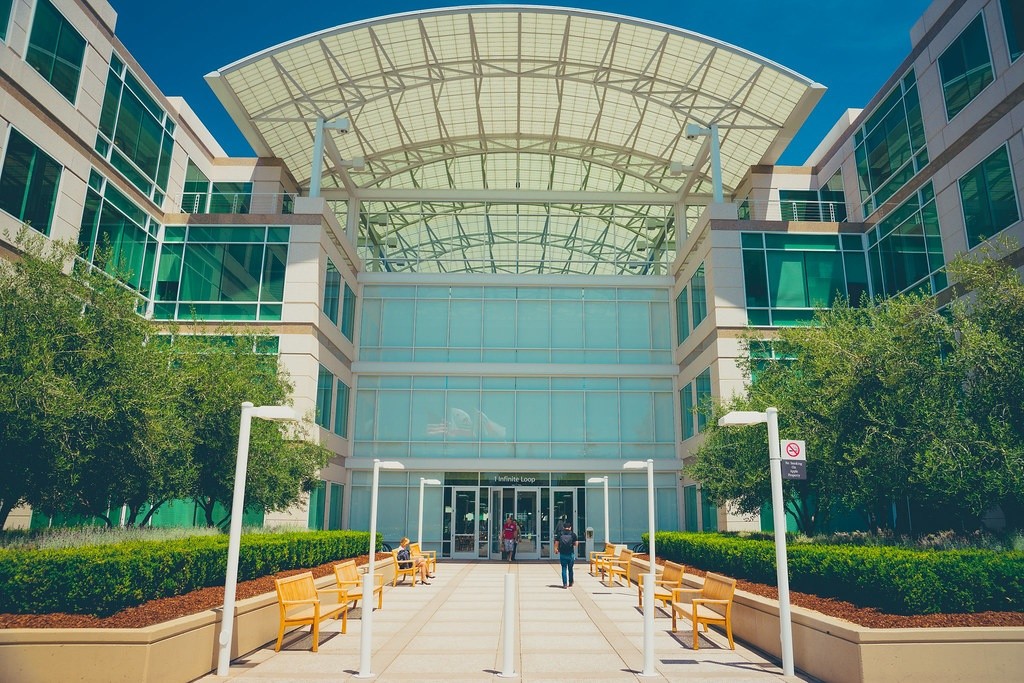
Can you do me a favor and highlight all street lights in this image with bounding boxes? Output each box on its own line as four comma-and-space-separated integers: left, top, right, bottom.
587, 475, 610, 543
622, 457, 661, 677
417, 477, 441, 551
717, 406, 797, 677
215, 401, 301, 677
354, 458, 405, 681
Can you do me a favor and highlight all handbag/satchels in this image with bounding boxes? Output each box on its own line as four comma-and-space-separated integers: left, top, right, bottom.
500, 543, 504, 552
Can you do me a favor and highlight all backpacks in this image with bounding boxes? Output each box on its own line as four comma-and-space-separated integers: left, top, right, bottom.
397, 550, 412, 569
559, 530, 573, 550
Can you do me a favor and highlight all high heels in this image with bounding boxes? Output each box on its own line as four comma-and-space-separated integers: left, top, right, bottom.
422, 580, 431, 585
426, 574, 435, 579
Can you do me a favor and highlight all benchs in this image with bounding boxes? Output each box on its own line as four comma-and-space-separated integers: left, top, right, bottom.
671, 571, 737, 650
333, 560, 384, 620
637, 560, 685, 619
391, 543, 436, 587
590, 543, 633, 588
274, 571, 349, 652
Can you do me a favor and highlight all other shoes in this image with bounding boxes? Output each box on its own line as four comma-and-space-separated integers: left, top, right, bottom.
569, 581, 573, 586
564, 585, 567, 589
507, 556, 512, 562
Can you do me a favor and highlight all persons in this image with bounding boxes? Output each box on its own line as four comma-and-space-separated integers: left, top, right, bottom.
554, 522, 579, 589
500, 517, 518, 562
396, 536, 435, 586
511, 520, 522, 560
